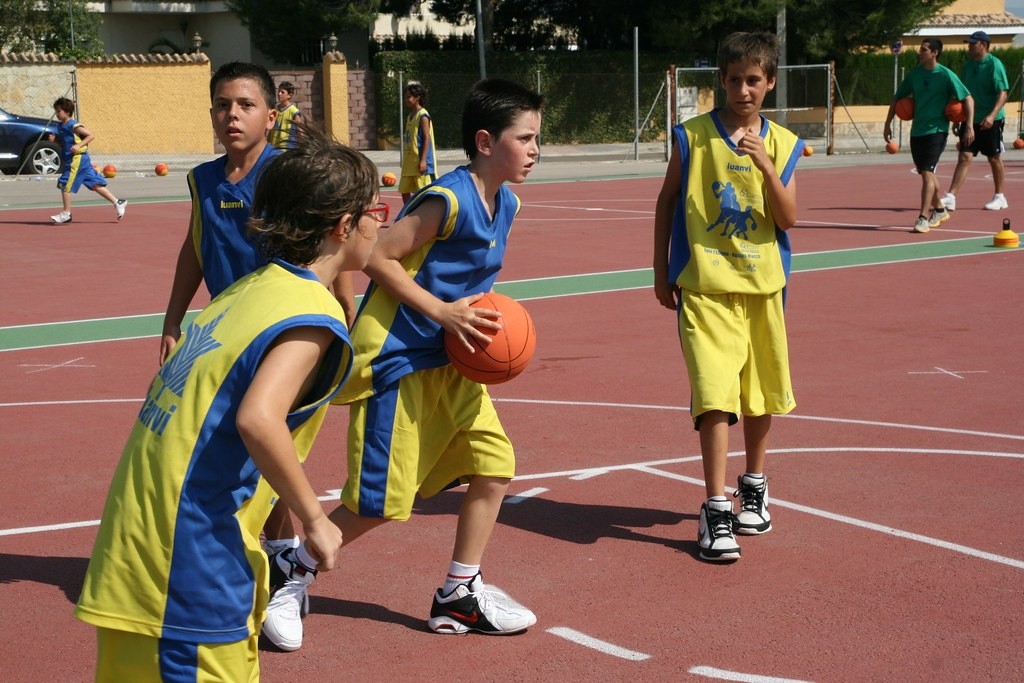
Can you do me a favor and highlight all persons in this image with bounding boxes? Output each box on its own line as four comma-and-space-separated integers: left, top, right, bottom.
260, 79, 547, 652
653, 32, 806, 560
398, 84, 438, 205
49, 97, 128, 223
940, 32, 1009, 211
74, 143, 390, 683
267, 82, 304, 153
159, 61, 355, 619
883, 39, 975, 232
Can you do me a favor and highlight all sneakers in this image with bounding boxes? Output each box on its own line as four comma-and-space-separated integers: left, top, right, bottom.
262, 535, 310, 617
928, 208, 950, 227
428, 573, 536, 634
263, 547, 317, 651
914, 217, 930, 233
985, 194, 1008, 211
50, 211, 72, 224
734, 474, 772, 534
941, 191, 956, 211
115, 199, 128, 221
697, 498, 742, 560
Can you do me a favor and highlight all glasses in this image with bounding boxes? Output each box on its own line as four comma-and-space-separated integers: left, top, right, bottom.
365, 203, 391, 222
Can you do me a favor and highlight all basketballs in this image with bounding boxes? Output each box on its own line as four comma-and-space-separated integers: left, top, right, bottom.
1013, 139, 1024, 148
103, 164, 116, 178
155, 163, 168, 176
956, 142, 960, 149
382, 172, 396, 186
895, 96, 915, 120
945, 100, 966, 123
886, 142, 898, 154
443, 291, 538, 386
93, 165, 102, 174
803, 146, 813, 156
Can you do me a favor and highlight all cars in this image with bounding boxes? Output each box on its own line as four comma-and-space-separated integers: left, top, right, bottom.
0, 108, 63, 175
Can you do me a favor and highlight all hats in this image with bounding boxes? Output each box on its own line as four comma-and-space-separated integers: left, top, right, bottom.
964, 31, 991, 44
